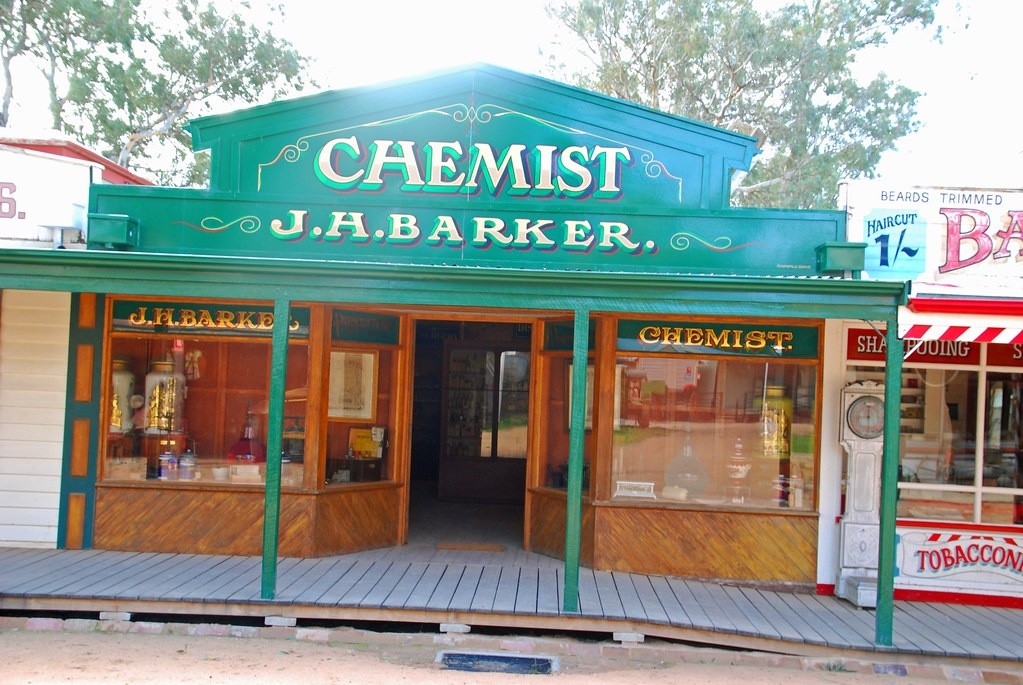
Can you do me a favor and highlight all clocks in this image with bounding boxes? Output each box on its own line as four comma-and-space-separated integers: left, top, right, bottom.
846, 395, 885, 439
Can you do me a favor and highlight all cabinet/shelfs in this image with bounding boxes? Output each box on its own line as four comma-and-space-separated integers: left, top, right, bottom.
437, 324, 530, 505
